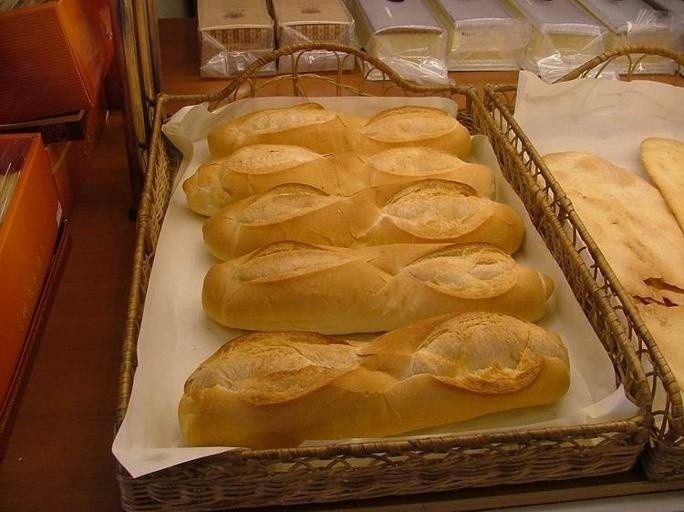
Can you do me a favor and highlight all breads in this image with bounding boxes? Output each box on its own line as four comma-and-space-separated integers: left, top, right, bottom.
201, 176, 526, 262
208, 102, 472, 160
178, 308, 570, 448
182, 143, 497, 218
200, 239, 555, 335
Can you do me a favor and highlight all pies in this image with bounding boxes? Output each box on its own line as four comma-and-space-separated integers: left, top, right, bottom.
640, 137, 683, 232
534, 151, 684, 397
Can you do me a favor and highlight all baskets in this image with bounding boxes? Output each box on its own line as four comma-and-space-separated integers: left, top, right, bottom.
108, 37, 654, 511
478, 32, 684, 481
